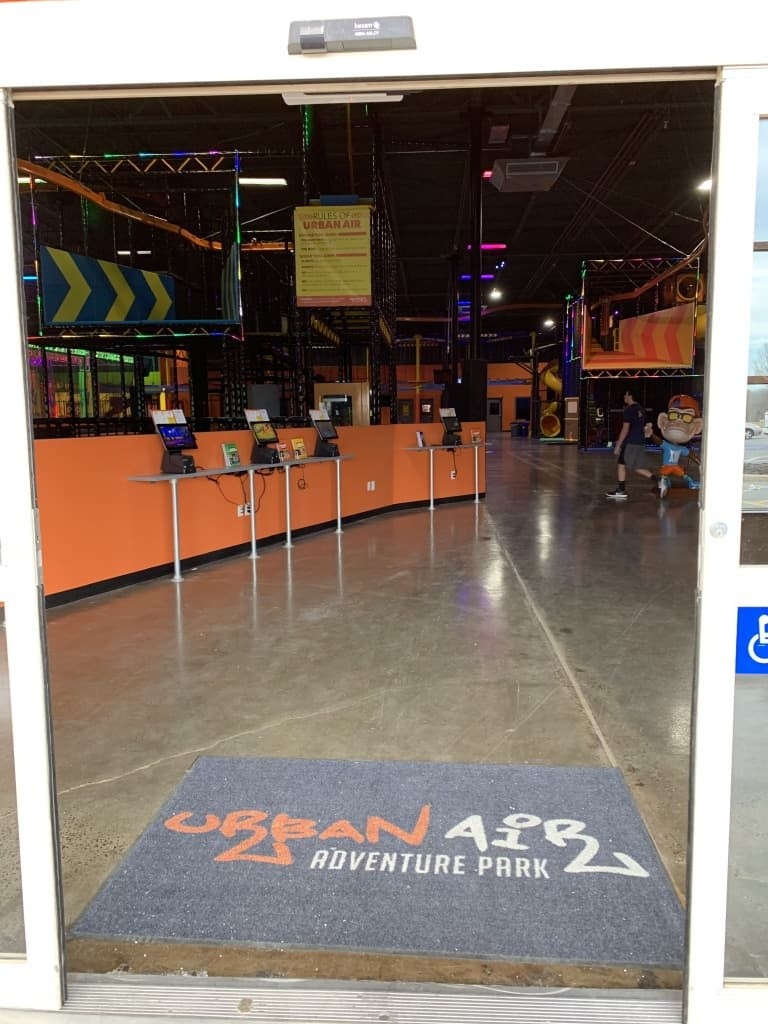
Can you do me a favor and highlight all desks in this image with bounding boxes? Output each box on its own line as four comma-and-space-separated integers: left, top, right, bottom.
128, 453, 353, 582
401, 442, 495, 510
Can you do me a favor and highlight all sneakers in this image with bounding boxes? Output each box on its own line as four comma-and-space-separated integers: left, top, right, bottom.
655, 474, 668, 498
606, 491, 628, 498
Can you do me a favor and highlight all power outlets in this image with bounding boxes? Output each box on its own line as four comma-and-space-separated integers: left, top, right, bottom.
451, 471, 456, 480
245, 503, 251, 516
238, 504, 244, 518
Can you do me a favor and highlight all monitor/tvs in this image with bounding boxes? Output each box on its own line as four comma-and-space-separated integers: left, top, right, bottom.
442, 416, 462, 433
249, 421, 279, 444
313, 419, 339, 440
157, 424, 198, 450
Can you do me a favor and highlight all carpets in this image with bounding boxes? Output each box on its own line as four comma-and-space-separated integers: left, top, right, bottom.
69, 754, 687, 970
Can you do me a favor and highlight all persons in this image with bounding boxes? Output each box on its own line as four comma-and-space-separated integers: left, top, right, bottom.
605, 391, 668, 499
643, 395, 703, 490
